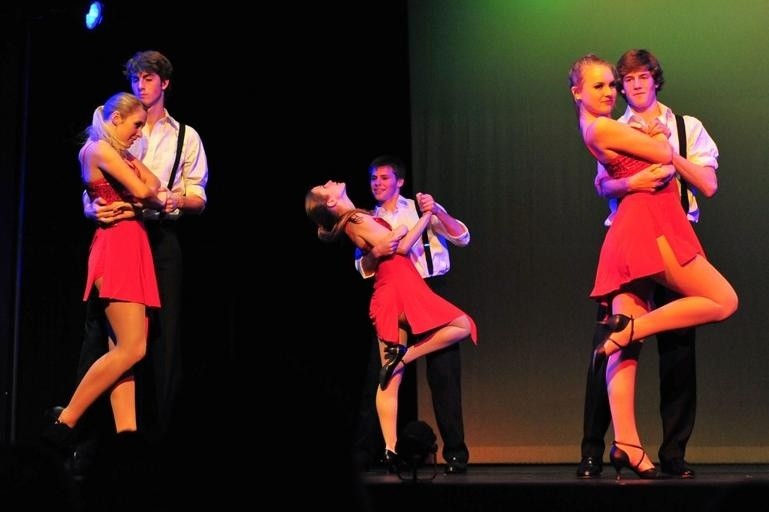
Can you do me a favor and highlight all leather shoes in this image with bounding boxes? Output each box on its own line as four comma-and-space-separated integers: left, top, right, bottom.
610, 446, 673, 480
46, 407, 81, 471
444, 456, 468, 474
663, 459, 696, 478
576, 456, 604, 478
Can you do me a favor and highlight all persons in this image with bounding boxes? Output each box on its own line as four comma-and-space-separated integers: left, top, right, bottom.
575, 49, 718, 480
352, 155, 471, 474
305, 180, 478, 479
52, 91, 170, 432
66, 50, 208, 434
569, 53, 740, 482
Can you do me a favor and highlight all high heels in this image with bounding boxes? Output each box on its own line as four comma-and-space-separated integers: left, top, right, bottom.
383, 449, 411, 474
380, 344, 407, 391
592, 314, 631, 384
